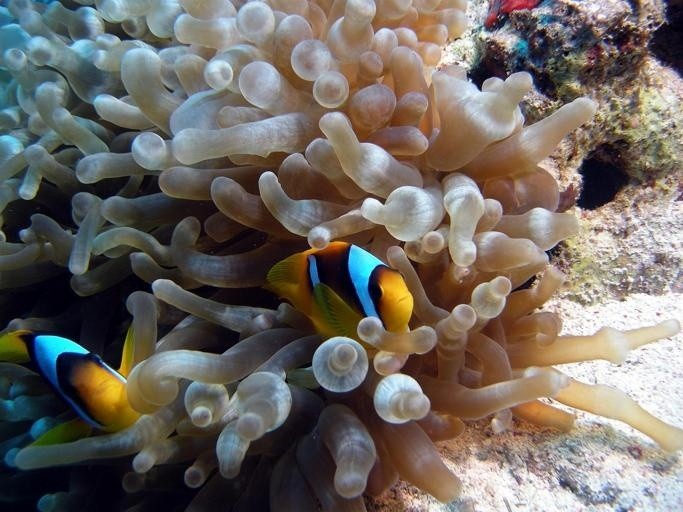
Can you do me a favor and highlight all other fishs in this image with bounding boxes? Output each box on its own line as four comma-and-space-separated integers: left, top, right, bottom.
0, 321, 143, 448
261, 241, 414, 349
